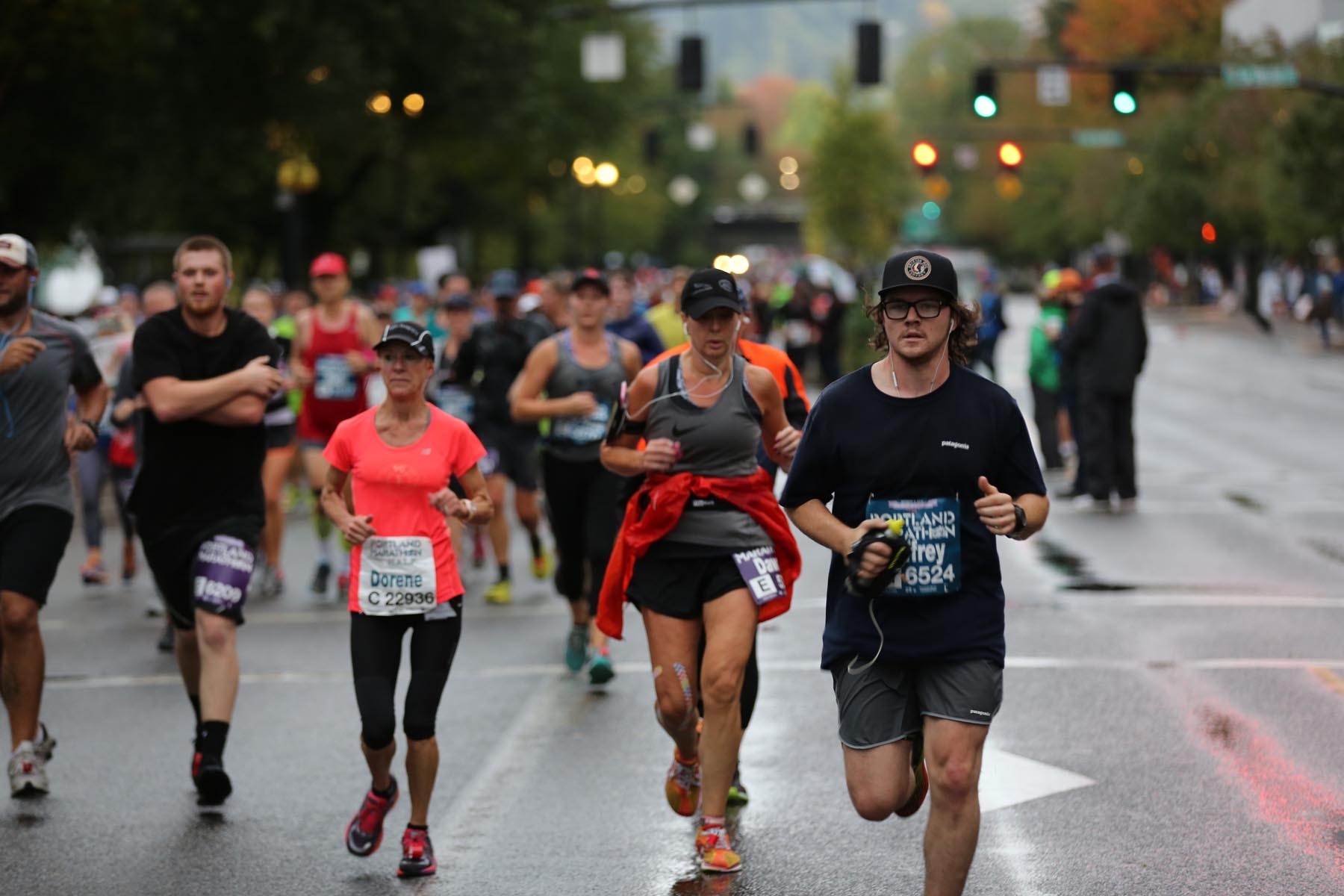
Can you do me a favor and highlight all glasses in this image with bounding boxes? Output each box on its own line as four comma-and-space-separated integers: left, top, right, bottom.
881, 297, 948, 320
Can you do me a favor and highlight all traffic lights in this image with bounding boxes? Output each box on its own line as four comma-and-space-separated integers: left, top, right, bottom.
968, 67, 999, 122
1198, 220, 1222, 247
1001, 141, 1019, 182
916, 142, 934, 176
1114, 71, 1139, 118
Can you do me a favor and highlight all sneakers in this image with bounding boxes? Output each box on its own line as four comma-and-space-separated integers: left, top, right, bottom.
695, 817, 743, 873
191, 750, 232, 806
33, 722, 57, 763
664, 716, 701, 816
895, 729, 929, 818
7, 753, 51, 798
397, 823, 437, 878
345, 775, 400, 857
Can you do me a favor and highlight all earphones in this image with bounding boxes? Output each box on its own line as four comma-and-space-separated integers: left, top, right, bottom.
30, 275, 35, 284
684, 322, 689, 334
741, 319, 746, 329
949, 318, 955, 334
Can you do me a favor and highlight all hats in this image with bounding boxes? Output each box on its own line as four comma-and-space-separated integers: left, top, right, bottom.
0, 233, 40, 277
490, 269, 519, 300
372, 322, 435, 362
736, 285, 748, 311
310, 252, 345, 276
878, 249, 959, 302
571, 269, 610, 296
680, 269, 741, 320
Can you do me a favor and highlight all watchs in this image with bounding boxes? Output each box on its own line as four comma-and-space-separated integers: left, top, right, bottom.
1006, 503, 1026, 540
459, 498, 476, 524
82, 418, 100, 437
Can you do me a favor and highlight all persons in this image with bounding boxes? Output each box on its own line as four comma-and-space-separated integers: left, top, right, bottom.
972, 237, 1344, 515
606, 259, 699, 364
320, 321, 496, 875
248, 247, 572, 601
597, 271, 812, 871
125, 235, 281, 809
779, 250, 1050, 896
509, 268, 640, 696
0, 233, 109, 801
39, 238, 180, 656
748, 249, 870, 392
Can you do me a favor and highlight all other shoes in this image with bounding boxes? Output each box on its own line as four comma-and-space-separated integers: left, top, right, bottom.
565, 621, 588, 670
80, 548, 107, 584
531, 538, 549, 577
1060, 487, 1136, 513
280, 484, 303, 513
589, 656, 613, 685
728, 760, 749, 802
337, 574, 350, 588
258, 569, 284, 595
158, 625, 176, 650
122, 548, 135, 577
311, 564, 331, 593
482, 577, 510, 603
